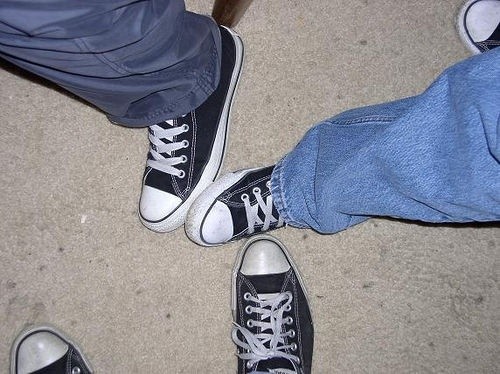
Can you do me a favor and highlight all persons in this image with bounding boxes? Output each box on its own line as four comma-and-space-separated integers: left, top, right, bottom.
184, 0, 500, 247
0, 0, 244, 233
10, 233, 314, 374
